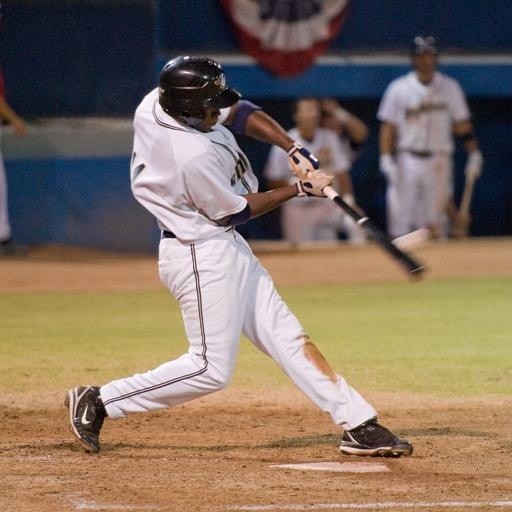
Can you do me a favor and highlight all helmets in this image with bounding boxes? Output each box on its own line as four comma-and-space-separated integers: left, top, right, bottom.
159, 55, 243, 130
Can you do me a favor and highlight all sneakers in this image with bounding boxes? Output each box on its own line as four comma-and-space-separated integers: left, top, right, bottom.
339, 415, 413, 457
64, 384, 109, 455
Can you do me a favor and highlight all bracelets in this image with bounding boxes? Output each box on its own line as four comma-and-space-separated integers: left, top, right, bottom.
342, 192, 356, 206
288, 176, 299, 185
333, 107, 350, 127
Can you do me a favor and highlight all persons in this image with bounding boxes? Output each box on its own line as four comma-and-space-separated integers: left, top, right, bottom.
64, 54, 413, 457
316, 94, 370, 245
0, 68, 28, 243
262, 96, 365, 243
377, 34, 485, 239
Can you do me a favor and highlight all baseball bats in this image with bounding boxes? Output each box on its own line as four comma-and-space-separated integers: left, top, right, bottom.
307, 168, 427, 282
